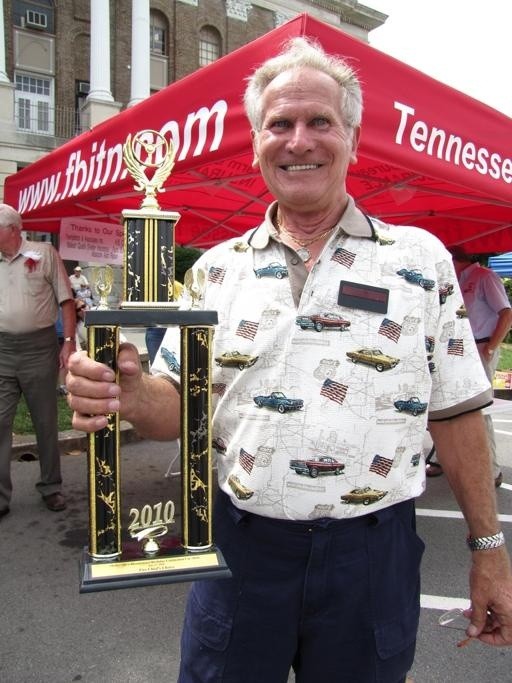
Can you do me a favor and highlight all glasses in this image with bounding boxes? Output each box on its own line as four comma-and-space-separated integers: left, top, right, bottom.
76, 303, 86, 312
81, 285, 86, 287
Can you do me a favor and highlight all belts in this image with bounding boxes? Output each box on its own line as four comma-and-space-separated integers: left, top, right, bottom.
475, 337, 491, 343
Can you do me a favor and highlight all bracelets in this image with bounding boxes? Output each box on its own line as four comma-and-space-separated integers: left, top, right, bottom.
466, 532, 506, 552
485, 347, 496, 355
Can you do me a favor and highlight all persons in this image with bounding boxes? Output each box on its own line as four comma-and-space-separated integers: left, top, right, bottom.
426, 245, 512, 486
68, 34, 512, 683
56, 266, 126, 395
0, 204, 76, 517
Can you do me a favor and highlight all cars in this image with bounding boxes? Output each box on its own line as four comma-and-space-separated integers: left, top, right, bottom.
212, 349, 386, 506
396, 269, 468, 318
159, 347, 180, 374
253, 262, 428, 417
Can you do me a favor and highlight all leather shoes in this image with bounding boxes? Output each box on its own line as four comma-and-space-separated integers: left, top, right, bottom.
42, 494, 67, 511
426, 461, 443, 477
495, 472, 504, 488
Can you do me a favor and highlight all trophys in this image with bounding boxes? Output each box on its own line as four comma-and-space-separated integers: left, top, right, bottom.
77, 129, 232, 594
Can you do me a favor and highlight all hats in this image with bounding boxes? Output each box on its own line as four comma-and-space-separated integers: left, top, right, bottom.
74, 267, 82, 271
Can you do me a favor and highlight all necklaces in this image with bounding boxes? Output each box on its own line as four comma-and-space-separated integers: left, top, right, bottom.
275, 220, 340, 262
